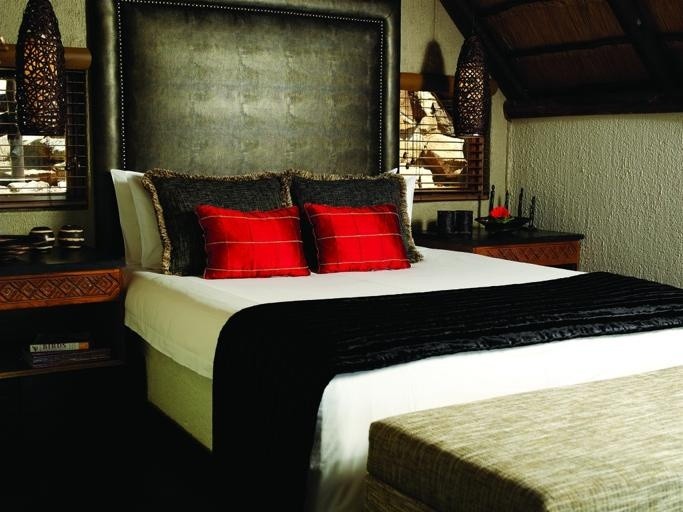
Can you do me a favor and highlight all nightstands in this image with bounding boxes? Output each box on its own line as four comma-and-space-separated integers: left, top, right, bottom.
420, 226, 586, 273
0, 245, 124, 381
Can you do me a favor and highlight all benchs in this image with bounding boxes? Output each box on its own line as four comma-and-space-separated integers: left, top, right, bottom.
362, 365, 683, 511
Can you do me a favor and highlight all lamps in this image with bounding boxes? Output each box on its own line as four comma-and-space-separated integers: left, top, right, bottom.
453, 0, 492, 140
15, 0, 67, 138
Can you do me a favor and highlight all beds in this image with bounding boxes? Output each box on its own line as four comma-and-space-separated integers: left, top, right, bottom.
87, 0, 683, 510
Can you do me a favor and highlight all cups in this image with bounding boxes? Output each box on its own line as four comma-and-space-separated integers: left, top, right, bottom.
437, 209, 473, 235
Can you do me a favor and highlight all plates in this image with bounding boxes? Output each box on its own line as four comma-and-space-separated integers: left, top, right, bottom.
471, 218, 531, 229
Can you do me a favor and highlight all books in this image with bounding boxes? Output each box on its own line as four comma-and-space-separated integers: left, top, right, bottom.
24, 340, 113, 369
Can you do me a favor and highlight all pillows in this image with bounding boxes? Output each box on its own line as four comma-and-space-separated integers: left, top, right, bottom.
284, 169, 420, 265
140, 169, 293, 275
197, 199, 311, 280
305, 200, 412, 274
